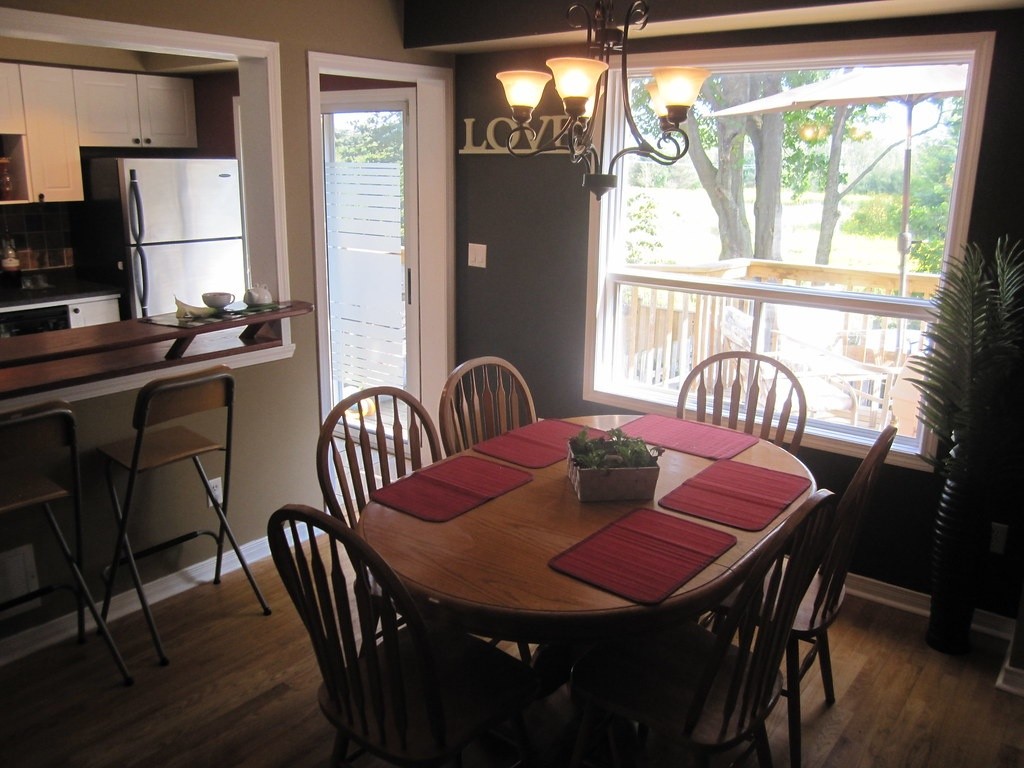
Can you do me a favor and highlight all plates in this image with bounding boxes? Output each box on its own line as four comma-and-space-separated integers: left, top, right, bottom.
222, 302, 248, 313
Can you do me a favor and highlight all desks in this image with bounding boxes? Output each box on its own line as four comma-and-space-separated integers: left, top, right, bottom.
358, 410, 812, 768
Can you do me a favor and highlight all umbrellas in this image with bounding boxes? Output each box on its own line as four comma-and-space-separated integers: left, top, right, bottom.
701, 62, 969, 432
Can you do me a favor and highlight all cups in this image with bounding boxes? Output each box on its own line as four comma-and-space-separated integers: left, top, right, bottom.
202, 291, 236, 308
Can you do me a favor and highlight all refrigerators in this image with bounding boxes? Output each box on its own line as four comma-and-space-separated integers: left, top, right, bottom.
80, 155, 248, 320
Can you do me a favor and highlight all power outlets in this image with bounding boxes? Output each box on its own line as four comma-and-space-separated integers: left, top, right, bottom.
207, 478, 223, 509
989, 520, 1005, 552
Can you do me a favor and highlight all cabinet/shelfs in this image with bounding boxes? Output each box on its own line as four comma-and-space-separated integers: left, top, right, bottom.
73, 68, 199, 145
0, 62, 25, 134
19, 64, 87, 202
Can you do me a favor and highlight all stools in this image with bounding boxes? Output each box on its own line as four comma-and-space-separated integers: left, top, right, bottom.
89, 361, 273, 662
0, 409, 134, 694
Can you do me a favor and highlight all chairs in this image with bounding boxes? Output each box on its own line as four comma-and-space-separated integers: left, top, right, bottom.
676, 349, 808, 455
267, 502, 543, 767
559, 490, 842, 768
697, 428, 900, 768
438, 356, 537, 459
316, 385, 531, 677
722, 305, 883, 426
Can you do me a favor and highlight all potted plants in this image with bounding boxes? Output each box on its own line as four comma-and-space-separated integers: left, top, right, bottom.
566, 429, 662, 502
926, 235, 1024, 652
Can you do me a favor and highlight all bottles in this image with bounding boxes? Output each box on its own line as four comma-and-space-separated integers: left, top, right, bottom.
0, 156, 16, 200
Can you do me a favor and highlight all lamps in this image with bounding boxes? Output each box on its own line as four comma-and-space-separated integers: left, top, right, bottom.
494, 1, 709, 203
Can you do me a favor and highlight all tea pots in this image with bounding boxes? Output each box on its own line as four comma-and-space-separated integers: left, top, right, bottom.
243, 283, 272, 304
